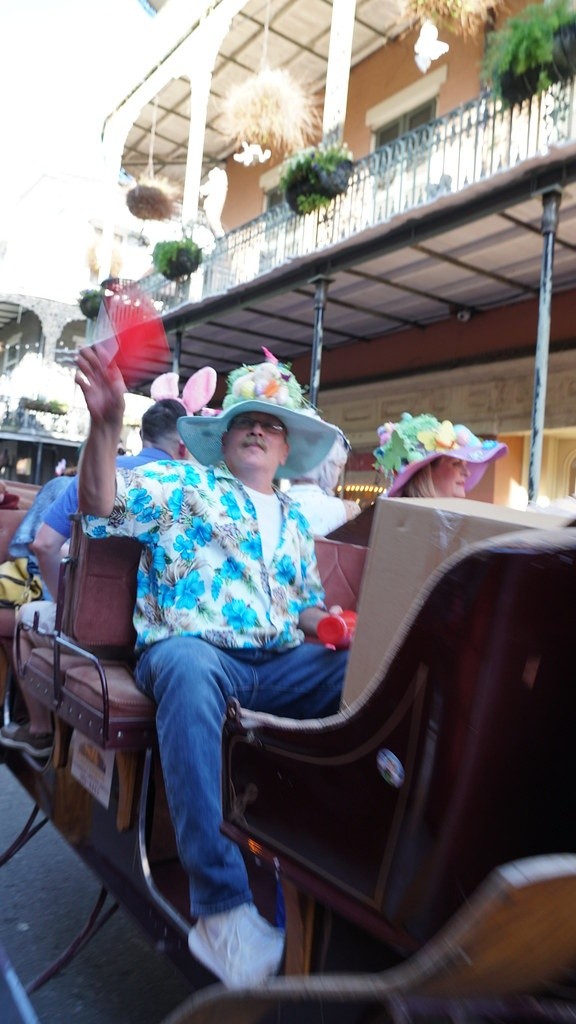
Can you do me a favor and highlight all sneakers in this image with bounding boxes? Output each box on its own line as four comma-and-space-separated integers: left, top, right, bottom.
188, 901, 285, 991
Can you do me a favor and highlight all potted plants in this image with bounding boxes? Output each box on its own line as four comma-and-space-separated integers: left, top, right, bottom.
151, 238, 202, 281
77, 290, 102, 320
478, 0, 576, 113
277, 142, 355, 216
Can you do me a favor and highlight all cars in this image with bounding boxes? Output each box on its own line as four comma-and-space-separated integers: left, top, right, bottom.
0, 397, 576, 1024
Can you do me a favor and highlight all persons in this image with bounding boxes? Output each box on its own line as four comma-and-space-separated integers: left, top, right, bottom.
374, 413, 508, 498
9, 365, 216, 756
74, 345, 350, 987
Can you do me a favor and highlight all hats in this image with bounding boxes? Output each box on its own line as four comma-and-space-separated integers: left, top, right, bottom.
176, 346, 352, 496
371, 412, 508, 497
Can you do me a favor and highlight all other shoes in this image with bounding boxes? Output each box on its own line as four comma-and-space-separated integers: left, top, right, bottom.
0, 721, 54, 757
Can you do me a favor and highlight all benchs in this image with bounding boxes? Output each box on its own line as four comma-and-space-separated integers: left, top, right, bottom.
1, 479, 576, 983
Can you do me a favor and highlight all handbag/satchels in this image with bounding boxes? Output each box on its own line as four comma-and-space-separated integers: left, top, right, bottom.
0, 555, 43, 609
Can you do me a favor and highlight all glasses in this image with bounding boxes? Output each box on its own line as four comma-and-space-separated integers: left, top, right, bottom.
231, 415, 287, 434
